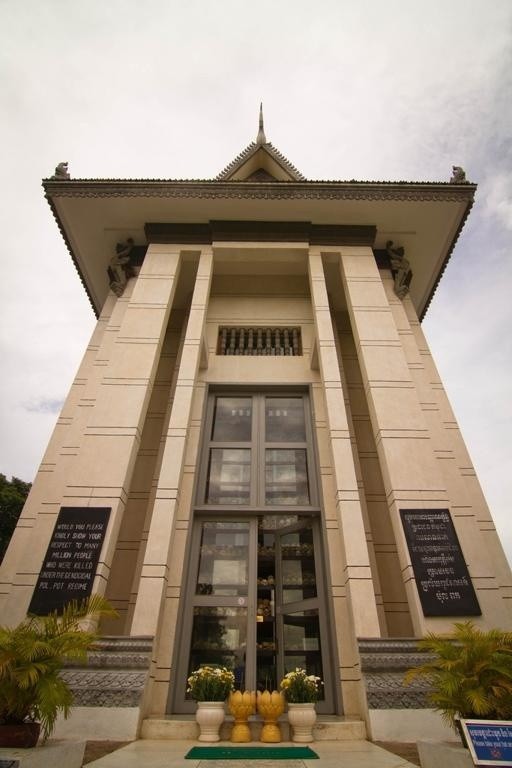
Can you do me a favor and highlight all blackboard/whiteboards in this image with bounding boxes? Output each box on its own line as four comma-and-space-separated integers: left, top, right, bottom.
27, 507, 113, 616
398, 508, 484, 617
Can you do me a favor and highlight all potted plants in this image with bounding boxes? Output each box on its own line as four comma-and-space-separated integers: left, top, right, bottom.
1, 593, 121, 749
403, 620, 512, 748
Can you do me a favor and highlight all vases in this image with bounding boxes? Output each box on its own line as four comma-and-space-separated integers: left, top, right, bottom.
195, 702, 225, 742
288, 703, 317, 742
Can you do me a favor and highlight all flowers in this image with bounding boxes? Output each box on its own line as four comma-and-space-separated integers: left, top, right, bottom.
279, 666, 325, 703
186, 664, 235, 701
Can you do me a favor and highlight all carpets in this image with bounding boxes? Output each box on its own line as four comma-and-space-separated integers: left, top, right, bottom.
184, 747, 319, 759
